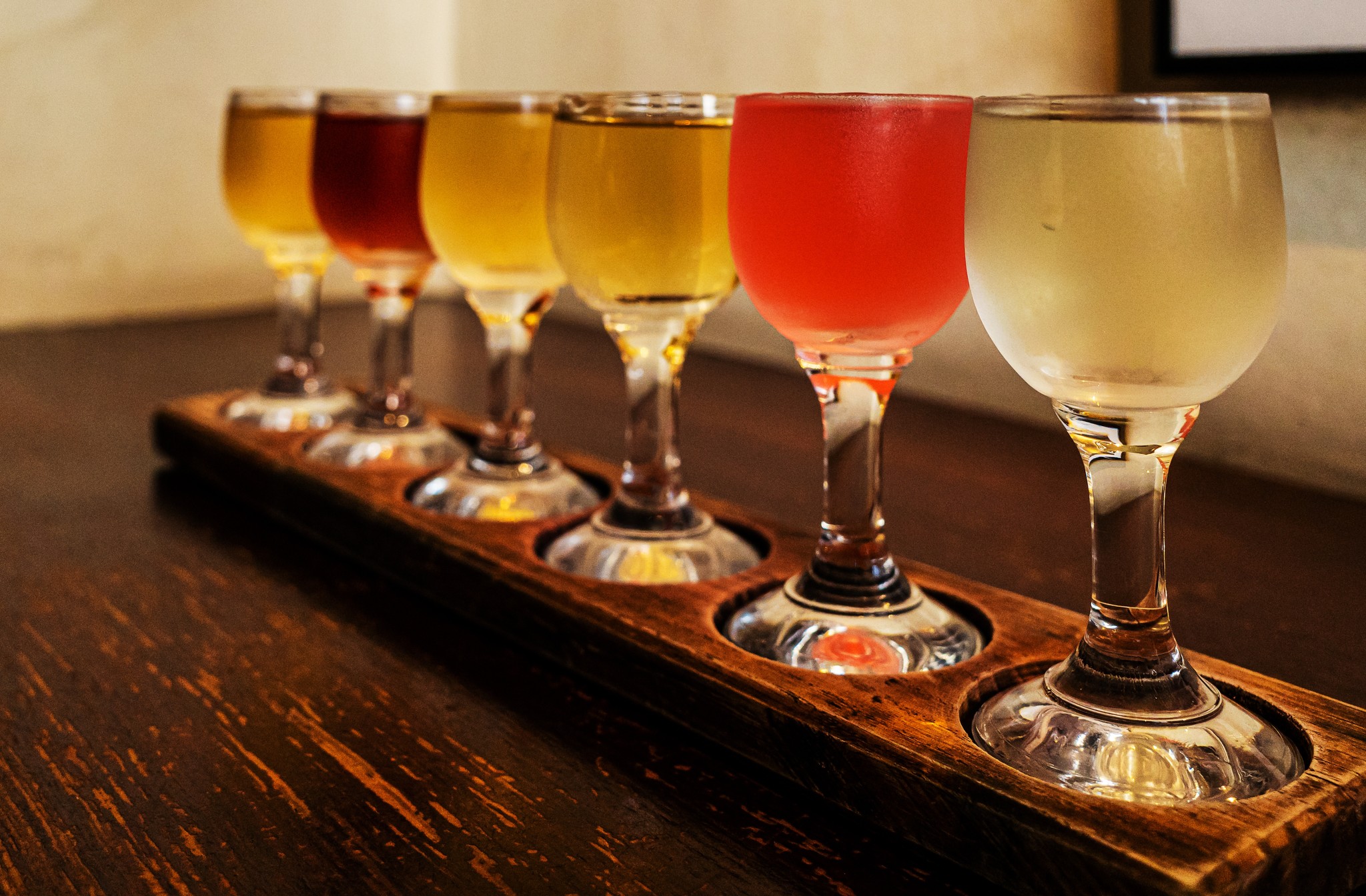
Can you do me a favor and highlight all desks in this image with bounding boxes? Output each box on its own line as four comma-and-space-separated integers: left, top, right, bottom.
2, 293, 1366, 896
